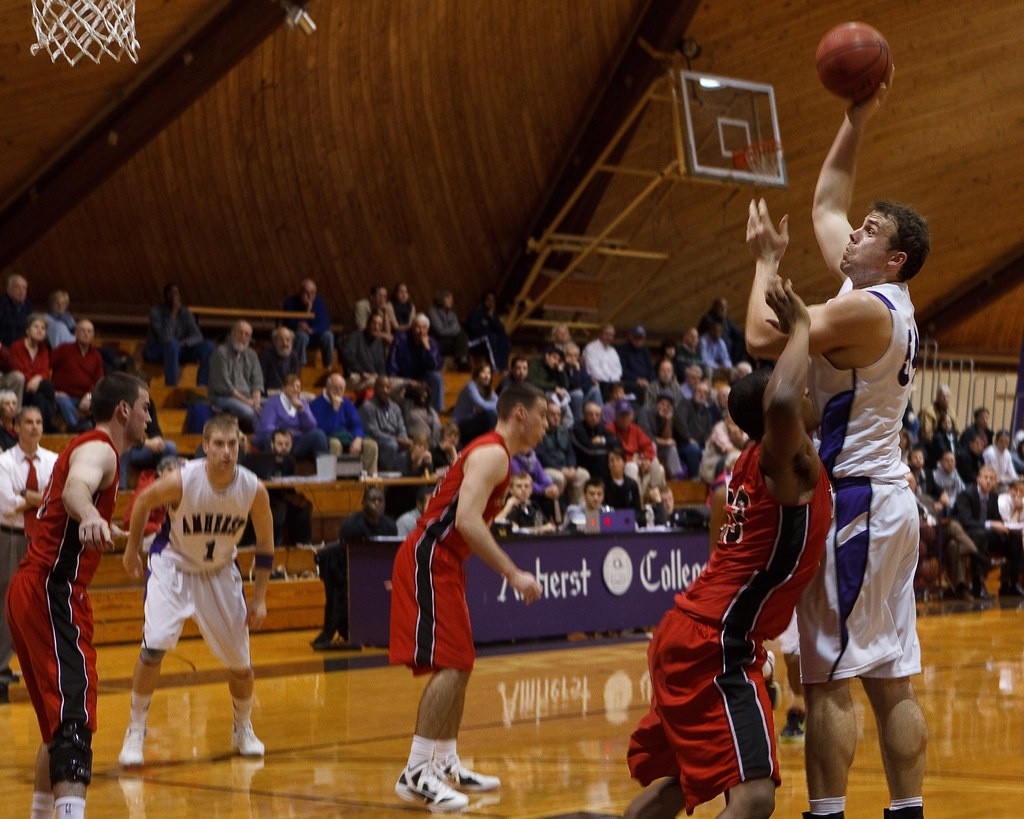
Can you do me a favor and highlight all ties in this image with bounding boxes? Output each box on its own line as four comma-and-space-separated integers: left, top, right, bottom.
23, 456, 41, 537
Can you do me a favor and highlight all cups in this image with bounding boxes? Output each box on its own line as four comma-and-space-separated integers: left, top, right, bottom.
317, 455, 338, 482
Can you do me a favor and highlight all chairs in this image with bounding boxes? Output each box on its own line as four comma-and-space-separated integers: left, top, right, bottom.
315, 544, 362, 650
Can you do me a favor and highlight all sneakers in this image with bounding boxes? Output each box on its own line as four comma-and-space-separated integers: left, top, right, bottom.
119, 727, 145, 766
762, 650, 782, 710
432, 753, 501, 790
779, 706, 807, 743
233, 719, 265, 756
395, 759, 469, 811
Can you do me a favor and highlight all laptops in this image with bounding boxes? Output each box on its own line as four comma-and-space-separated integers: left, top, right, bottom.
601, 509, 636, 535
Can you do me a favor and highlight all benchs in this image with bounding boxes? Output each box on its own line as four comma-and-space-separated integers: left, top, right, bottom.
41, 304, 1003, 644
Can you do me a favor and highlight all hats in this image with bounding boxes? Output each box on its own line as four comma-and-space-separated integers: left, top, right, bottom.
543, 344, 566, 360
656, 389, 676, 405
615, 399, 633, 413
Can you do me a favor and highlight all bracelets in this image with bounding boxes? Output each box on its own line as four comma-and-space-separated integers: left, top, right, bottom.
20, 490, 27, 497
83, 393, 92, 400
254, 552, 274, 570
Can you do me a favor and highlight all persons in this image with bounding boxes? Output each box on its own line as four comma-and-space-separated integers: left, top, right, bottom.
390, 382, 547, 813
0, 278, 1024, 706
4, 373, 152, 819
626, 277, 833, 819
744, 52, 932, 819
118, 415, 274, 768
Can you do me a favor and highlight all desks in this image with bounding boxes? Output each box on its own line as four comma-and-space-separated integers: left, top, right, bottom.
351, 524, 710, 650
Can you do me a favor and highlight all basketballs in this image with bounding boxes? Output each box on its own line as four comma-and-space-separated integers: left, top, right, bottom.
815, 20, 891, 101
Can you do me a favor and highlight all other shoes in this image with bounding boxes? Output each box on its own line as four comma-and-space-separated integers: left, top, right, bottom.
998, 582, 1024, 596
970, 582, 995, 601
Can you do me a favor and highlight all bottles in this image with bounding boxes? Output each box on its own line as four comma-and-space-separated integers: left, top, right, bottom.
535, 511, 543, 535
646, 505, 654, 530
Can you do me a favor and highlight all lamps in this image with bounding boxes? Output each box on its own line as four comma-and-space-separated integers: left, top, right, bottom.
286, 8, 317, 36
